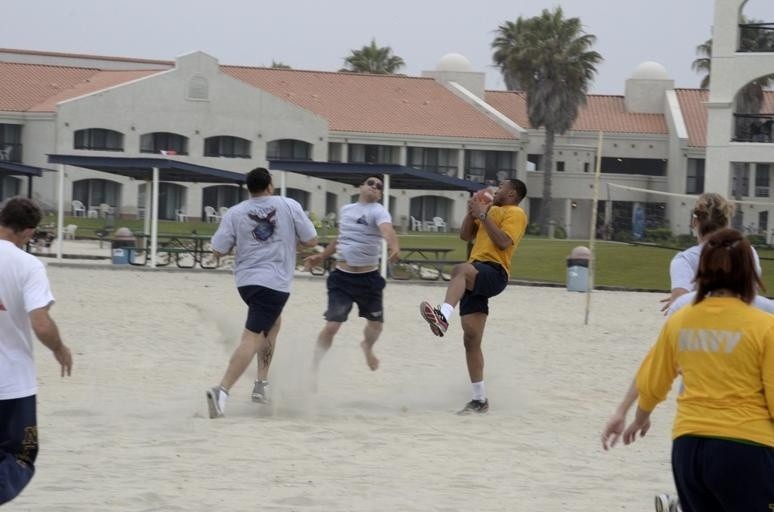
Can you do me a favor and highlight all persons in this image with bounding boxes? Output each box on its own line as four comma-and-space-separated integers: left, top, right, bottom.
299, 175, 401, 371
419, 177, 530, 414
0, 192, 72, 502
659, 192, 762, 317
203, 166, 319, 419
600, 290, 774, 512
623, 228, 774, 512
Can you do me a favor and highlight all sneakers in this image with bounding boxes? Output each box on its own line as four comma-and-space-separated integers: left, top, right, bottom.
453, 399, 490, 418
418, 300, 449, 338
205, 383, 230, 419
250, 376, 270, 404
654, 489, 684, 512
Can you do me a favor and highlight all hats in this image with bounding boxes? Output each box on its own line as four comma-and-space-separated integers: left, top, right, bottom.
245, 166, 273, 192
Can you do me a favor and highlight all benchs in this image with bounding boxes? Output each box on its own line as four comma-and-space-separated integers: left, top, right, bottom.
121, 231, 467, 281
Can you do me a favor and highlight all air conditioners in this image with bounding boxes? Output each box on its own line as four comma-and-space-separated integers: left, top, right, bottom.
469, 174, 481, 184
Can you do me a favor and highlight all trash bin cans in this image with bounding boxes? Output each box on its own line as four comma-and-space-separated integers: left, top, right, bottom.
111, 226, 136, 264
566, 246, 592, 291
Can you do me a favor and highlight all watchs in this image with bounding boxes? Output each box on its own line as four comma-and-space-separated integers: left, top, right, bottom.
478, 213, 488, 221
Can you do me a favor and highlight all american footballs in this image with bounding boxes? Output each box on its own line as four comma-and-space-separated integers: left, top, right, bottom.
472, 188, 495, 219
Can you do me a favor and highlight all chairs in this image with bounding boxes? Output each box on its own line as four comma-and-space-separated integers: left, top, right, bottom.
433, 216, 446, 233
321, 212, 336, 228
72, 200, 85, 217
175, 208, 188, 224
204, 206, 219, 224
100, 203, 115, 218
497, 171, 508, 183
220, 207, 229, 216
63, 224, 77, 240
411, 215, 421, 231
